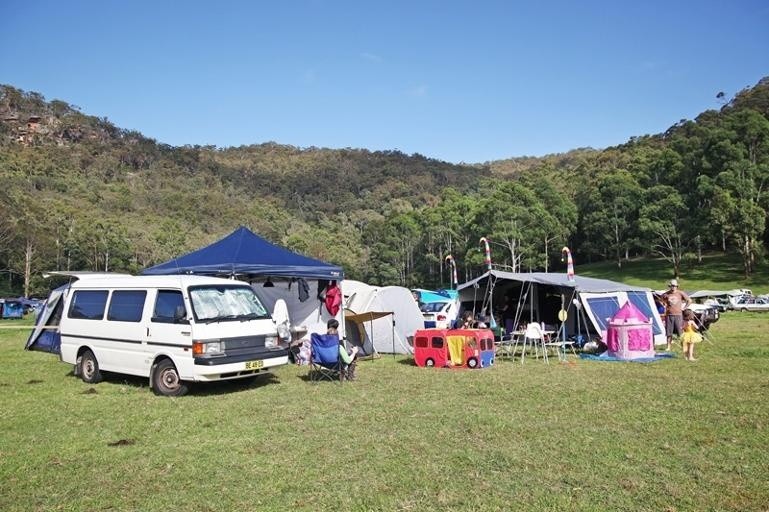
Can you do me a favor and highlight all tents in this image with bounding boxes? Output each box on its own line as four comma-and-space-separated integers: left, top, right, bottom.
24, 270, 72, 355
249, 278, 426, 360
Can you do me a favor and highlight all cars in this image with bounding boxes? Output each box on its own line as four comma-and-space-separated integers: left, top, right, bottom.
420, 300, 459, 330
681, 289, 769, 331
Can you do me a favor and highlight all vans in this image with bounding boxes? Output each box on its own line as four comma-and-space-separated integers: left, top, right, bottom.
42, 270, 289, 396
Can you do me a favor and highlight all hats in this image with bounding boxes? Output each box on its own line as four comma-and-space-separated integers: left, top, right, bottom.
668, 279, 679, 288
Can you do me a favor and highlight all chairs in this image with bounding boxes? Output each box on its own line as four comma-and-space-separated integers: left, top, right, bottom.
490, 318, 577, 364
309, 332, 357, 388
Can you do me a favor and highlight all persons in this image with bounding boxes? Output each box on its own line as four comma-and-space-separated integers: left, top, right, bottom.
326, 319, 339, 380
661, 280, 703, 361
339, 332, 358, 380
